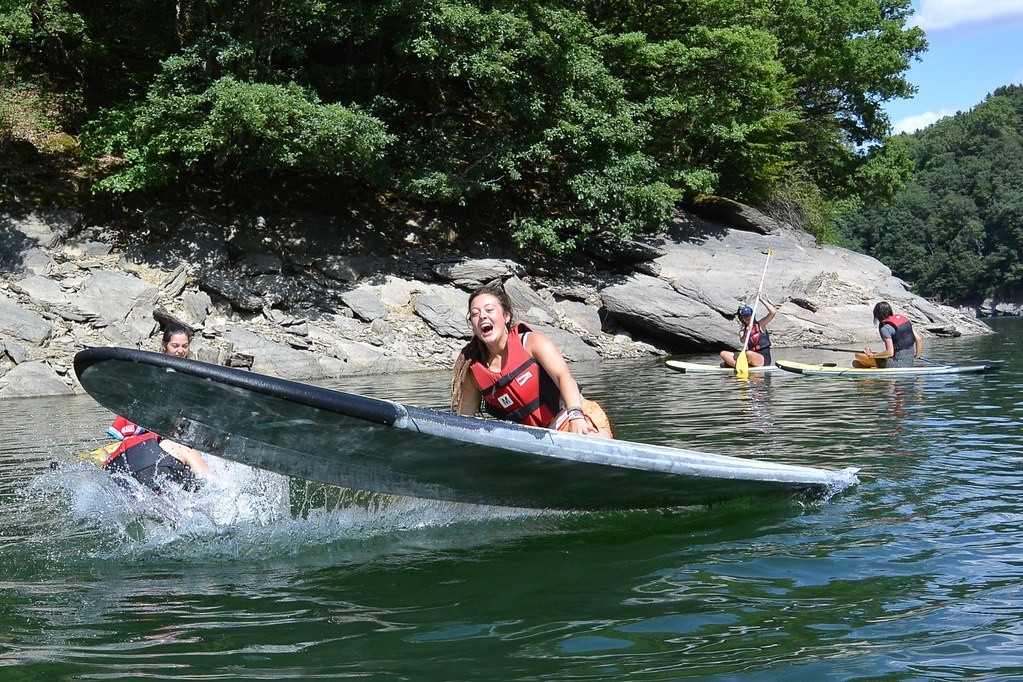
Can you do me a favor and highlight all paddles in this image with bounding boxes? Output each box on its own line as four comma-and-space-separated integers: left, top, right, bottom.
77, 439, 121, 466
734, 247, 773, 379
802, 343, 926, 361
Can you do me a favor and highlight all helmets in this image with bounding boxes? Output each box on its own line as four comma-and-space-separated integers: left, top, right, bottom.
740, 307, 753, 316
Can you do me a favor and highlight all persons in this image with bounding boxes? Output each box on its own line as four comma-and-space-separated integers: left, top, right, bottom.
853, 302, 922, 368
451, 287, 610, 439
162, 324, 191, 358
101, 431, 210, 496
720, 292, 776, 368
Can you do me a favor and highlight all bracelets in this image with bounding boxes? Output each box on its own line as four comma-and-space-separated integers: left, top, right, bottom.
873, 354, 874, 358
567, 406, 584, 420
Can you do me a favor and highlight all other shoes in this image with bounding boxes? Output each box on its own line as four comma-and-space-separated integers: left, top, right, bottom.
720, 362, 733, 368
852, 361, 870, 369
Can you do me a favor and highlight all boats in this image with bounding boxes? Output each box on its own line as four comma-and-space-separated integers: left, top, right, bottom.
80, 432, 208, 493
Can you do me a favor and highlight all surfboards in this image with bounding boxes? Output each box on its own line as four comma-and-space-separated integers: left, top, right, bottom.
68, 341, 867, 515
664, 360, 838, 375
774, 357, 1005, 376
